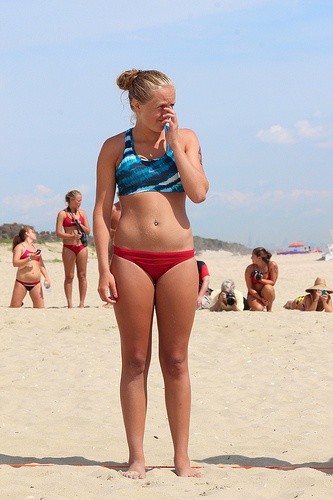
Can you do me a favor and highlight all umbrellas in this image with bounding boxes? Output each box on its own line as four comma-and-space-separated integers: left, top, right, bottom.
288, 242, 304, 250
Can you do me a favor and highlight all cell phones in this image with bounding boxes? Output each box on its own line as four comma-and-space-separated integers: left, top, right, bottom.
36, 250, 41, 255
322, 290, 327, 295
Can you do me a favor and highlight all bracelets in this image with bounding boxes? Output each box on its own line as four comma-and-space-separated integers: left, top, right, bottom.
28, 255, 32, 261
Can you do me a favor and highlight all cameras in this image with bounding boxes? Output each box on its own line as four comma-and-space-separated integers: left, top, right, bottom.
250, 270, 263, 280
79, 231, 87, 247
226, 292, 236, 305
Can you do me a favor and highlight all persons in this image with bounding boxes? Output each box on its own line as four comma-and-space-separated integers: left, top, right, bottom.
210, 279, 251, 311
10, 227, 50, 308
197, 260, 214, 311
283, 277, 333, 313
55, 189, 90, 309
245, 248, 280, 311
103, 200, 122, 310
93, 69, 211, 482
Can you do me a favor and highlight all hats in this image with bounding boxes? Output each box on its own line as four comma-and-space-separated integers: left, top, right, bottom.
306, 278, 333, 293
